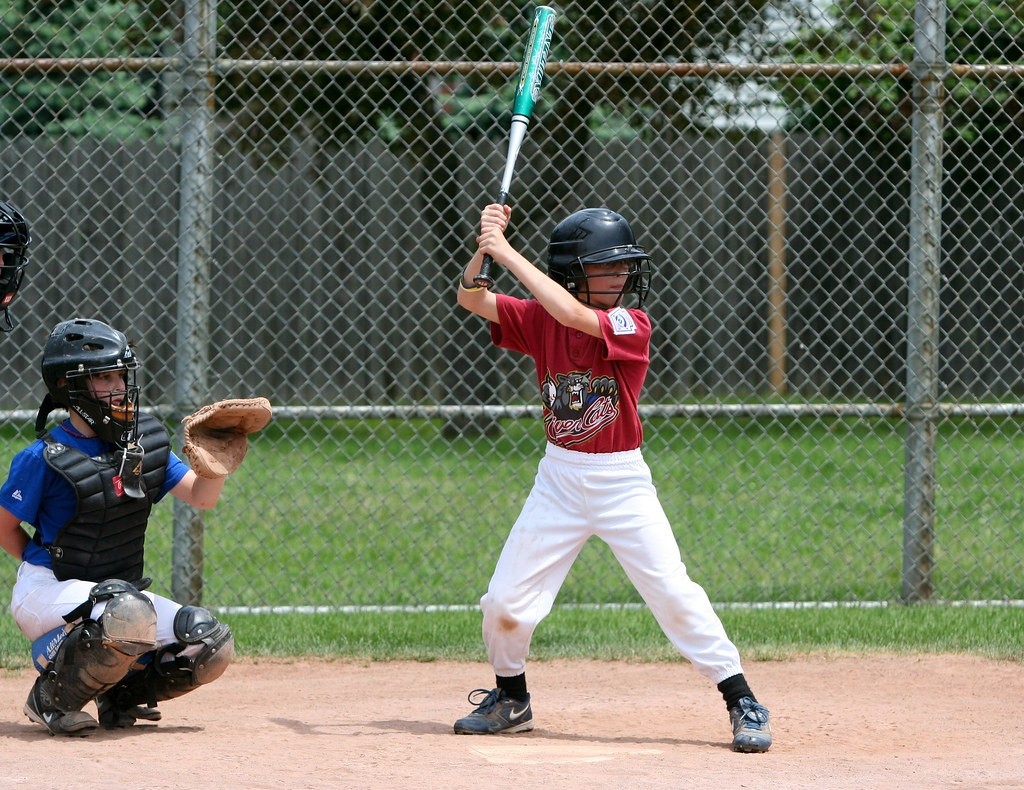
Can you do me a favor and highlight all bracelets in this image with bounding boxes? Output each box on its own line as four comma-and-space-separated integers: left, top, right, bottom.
459, 266, 483, 292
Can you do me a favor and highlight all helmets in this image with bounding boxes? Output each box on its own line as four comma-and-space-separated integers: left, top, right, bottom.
547, 207, 652, 293
0, 201, 30, 311
41, 318, 136, 443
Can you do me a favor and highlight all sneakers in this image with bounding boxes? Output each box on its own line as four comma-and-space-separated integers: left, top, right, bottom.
23, 676, 98, 736
94, 694, 162, 726
729, 696, 772, 753
454, 687, 533, 734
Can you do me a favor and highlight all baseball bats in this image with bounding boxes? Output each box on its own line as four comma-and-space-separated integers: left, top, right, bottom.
472, 5, 558, 288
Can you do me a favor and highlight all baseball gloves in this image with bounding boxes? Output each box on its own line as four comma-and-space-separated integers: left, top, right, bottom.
181, 397, 273, 480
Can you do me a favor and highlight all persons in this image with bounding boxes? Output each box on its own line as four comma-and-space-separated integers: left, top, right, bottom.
452, 205, 772, 754
0, 318, 273, 737
0, 201, 32, 332
